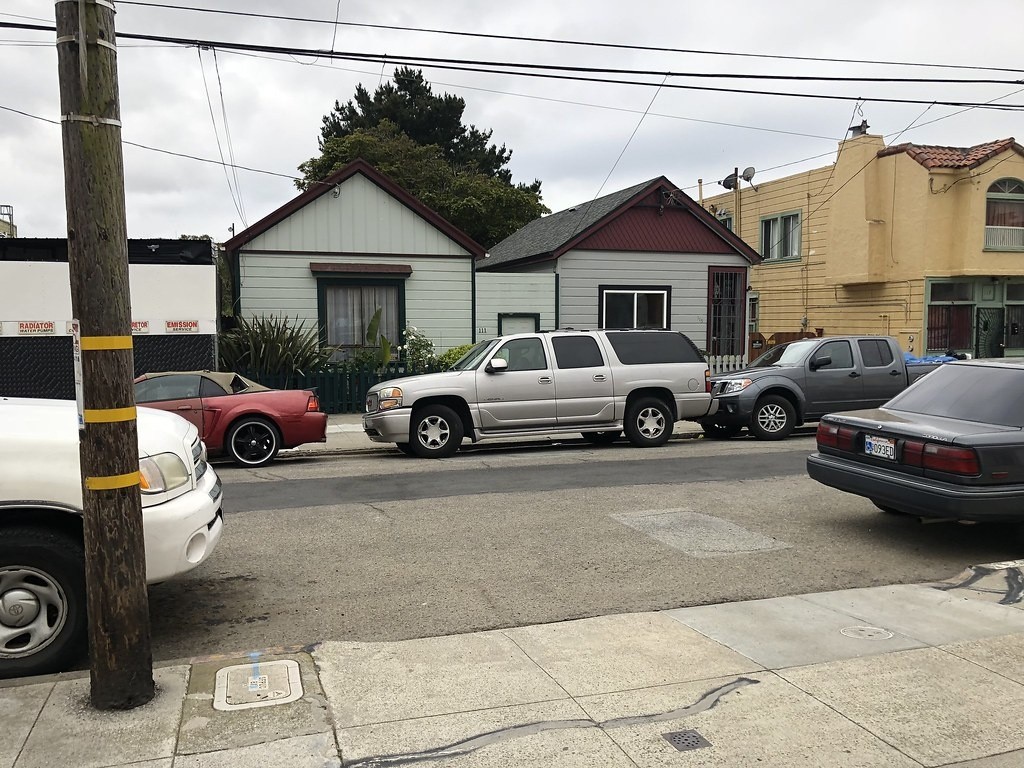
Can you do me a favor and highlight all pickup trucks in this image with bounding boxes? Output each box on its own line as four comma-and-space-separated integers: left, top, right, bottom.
360, 326, 719, 460
699, 334, 956, 441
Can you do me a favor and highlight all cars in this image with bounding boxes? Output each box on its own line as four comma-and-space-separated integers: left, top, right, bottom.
0, 395, 225, 679
806, 357, 1024, 525
132, 368, 329, 468
